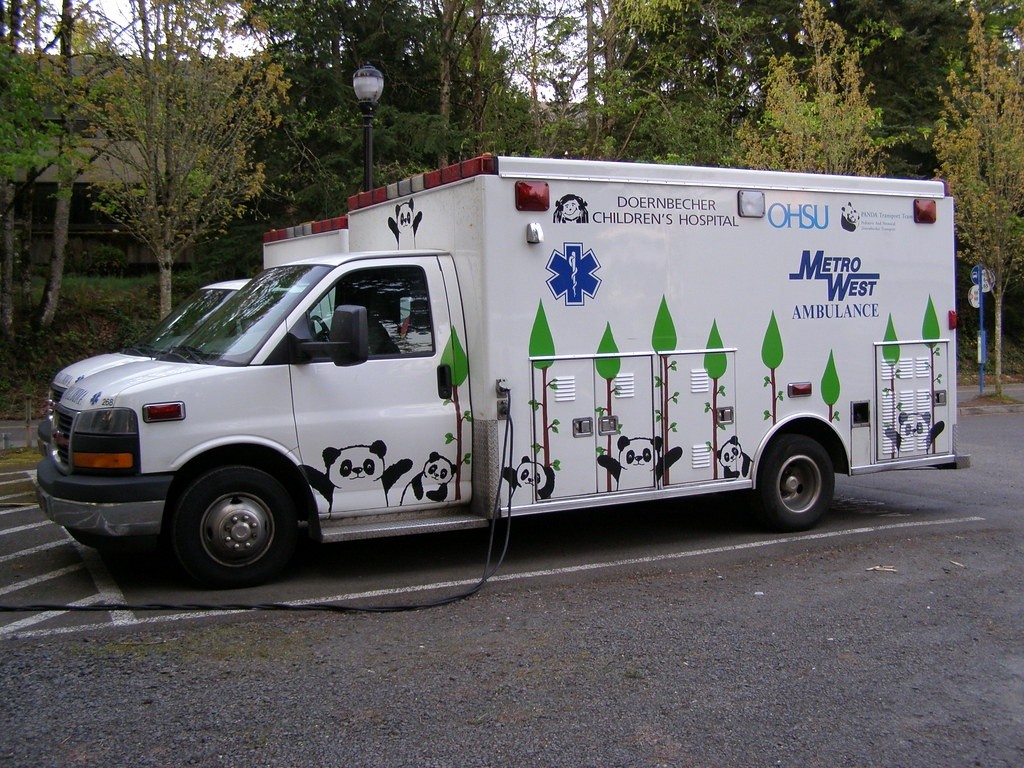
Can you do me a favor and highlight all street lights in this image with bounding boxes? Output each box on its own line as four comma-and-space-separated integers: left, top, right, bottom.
352, 62, 384, 193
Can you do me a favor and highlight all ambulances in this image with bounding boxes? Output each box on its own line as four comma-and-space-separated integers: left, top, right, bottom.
38, 214, 350, 472
37, 153, 972, 591
